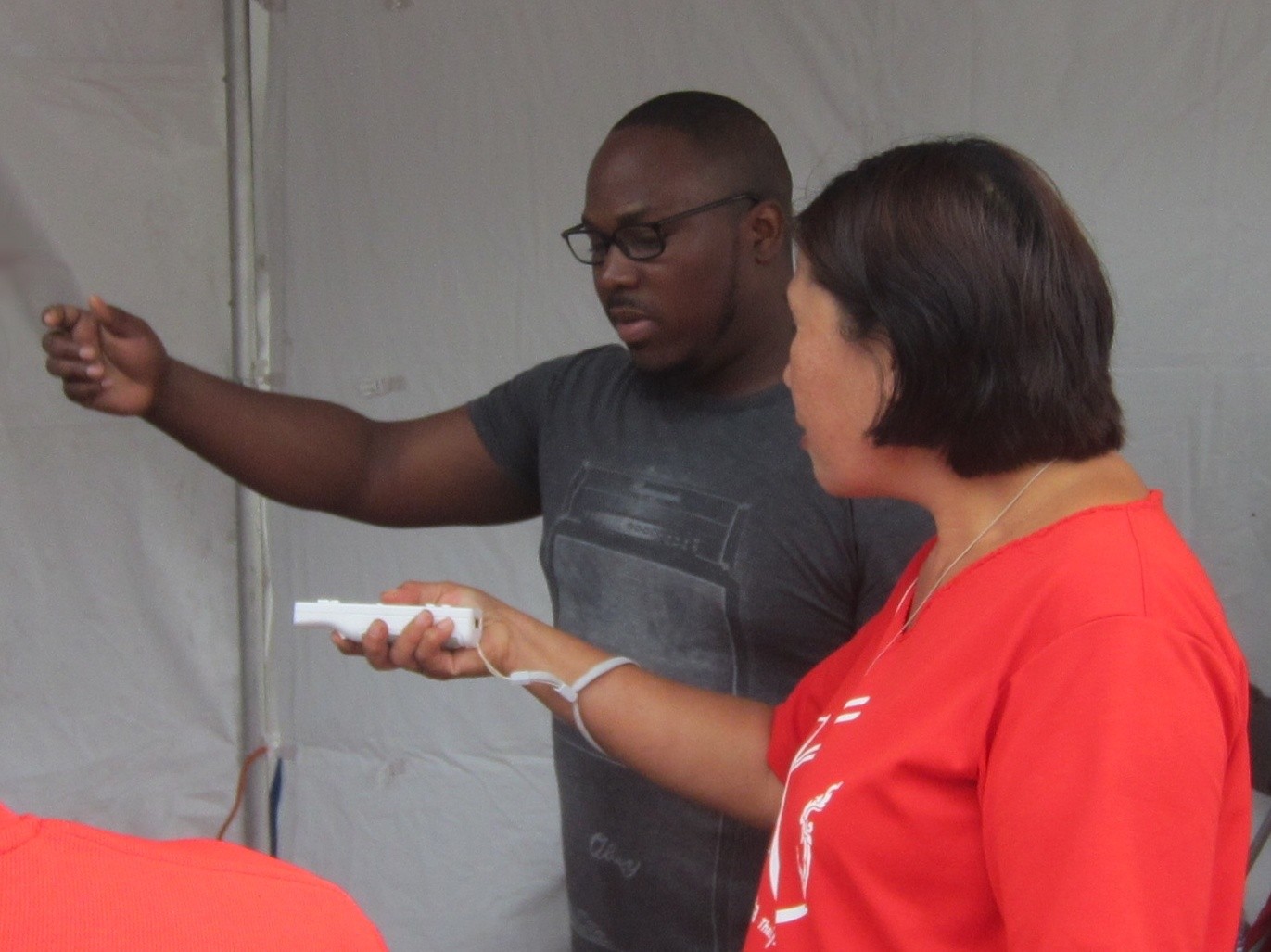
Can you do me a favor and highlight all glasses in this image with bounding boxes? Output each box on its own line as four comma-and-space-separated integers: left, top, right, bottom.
561, 195, 763, 265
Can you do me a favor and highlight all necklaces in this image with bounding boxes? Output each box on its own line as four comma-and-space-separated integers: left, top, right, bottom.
865, 458, 1055, 677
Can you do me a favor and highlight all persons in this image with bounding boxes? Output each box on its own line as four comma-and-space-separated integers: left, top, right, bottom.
42, 91, 937, 952
331, 138, 1252, 952
